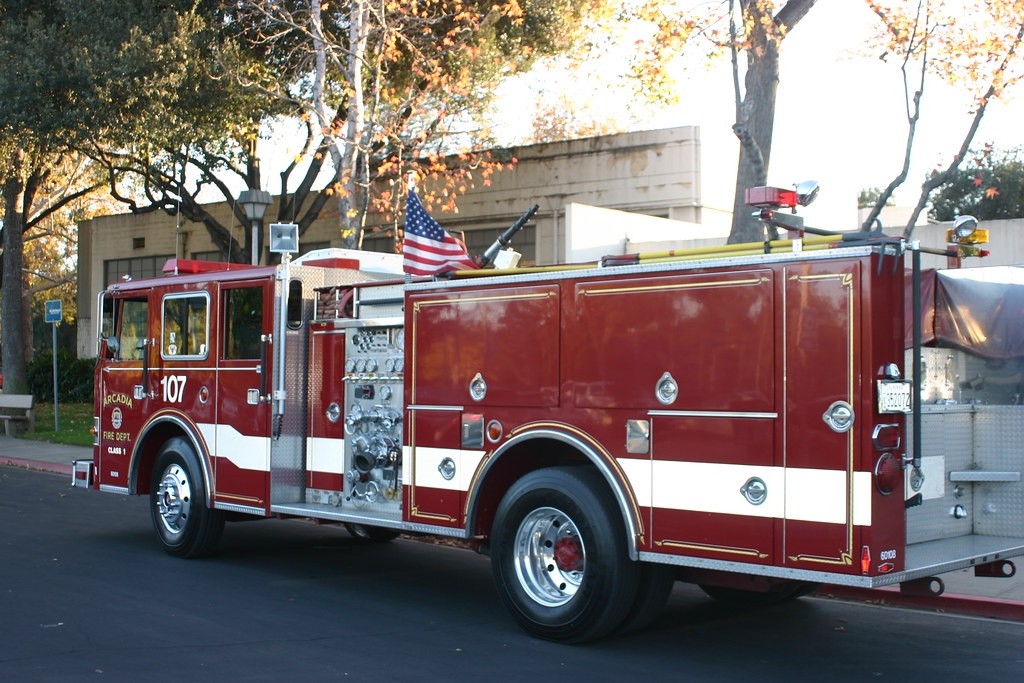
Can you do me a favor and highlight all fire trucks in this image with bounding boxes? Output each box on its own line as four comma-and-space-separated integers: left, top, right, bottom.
71, 179, 1023, 647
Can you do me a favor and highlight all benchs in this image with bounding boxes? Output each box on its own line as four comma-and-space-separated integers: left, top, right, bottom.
0, 393, 35, 438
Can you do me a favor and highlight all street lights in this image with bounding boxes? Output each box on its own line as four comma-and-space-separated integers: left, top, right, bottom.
236, 187, 274, 268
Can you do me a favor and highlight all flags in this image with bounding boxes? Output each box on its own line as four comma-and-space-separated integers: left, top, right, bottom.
402, 185, 482, 276
